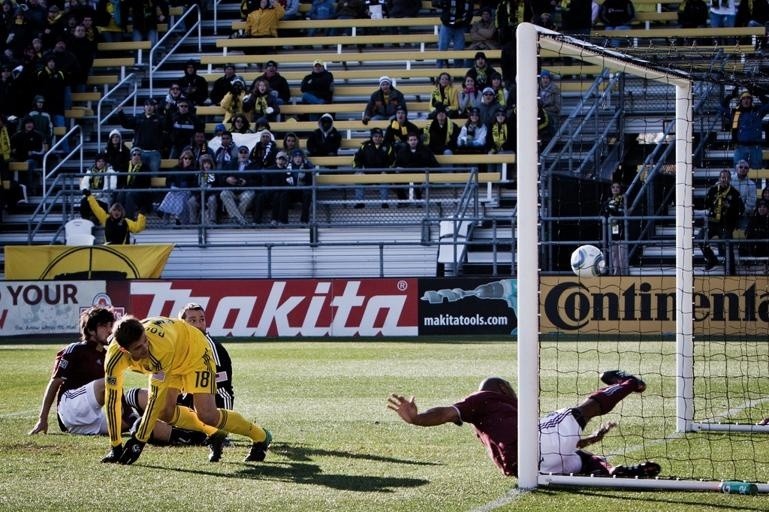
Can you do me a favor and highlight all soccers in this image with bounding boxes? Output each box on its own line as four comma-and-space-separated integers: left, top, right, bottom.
570, 245, 605, 278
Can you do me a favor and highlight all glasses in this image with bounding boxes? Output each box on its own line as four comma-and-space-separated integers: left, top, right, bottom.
132, 153, 142, 156
182, 156, 192, 161
483, 92, 495, 98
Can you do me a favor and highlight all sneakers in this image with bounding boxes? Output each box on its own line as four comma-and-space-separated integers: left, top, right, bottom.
244, 427, 274, 463
600, 368, 646, 395
610, 461, 664, 479
205, 428, 228, 463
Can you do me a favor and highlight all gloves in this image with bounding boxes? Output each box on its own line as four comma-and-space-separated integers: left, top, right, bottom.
118, 436, 148, 466
81, 188, 92, 197
100, 442, 124, 465
138, 207, 146, 214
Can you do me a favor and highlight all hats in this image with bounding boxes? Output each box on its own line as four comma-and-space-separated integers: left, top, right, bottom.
291, 148, 304, 157
739, 92, 754, 100
275, 150, 289, 159
312, 58, 324, 68
481, 86, 496, 95
494, 107, 506, 114
214, 123, 226, 132
539, 69, 550, 78
378, 76, 392, 87
469, 107, 480, 114
107, 128, 123, 140
260, 129, 271, 140
230, 75, 247, 88
237, 145, 249, 152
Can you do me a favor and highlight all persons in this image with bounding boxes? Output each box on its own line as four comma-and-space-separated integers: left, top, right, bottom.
124, 303, 235, 446
1, 1, 768, 276
99, 314, 273, 466
28, 305, 139, 436
386, 370, 661, 479
